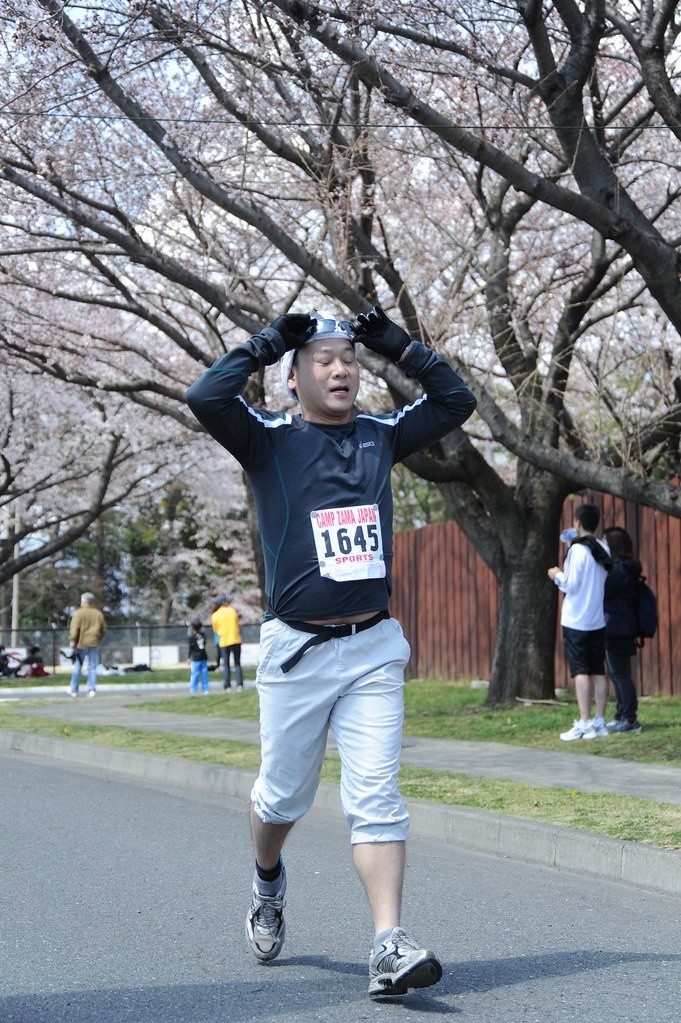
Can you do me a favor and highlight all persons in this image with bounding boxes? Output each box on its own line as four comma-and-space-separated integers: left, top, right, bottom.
547, 504, 611, 741
601, 526, 643, 735
211, 595, 243, 692
187, 622, 208, 697
185, 309, 477, 994
0, 645, 52, 678
65, 592, 107, 697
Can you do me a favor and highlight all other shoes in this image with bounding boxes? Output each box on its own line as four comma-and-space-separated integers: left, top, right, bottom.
86, 689, 95, 696
66, 688, 77, 697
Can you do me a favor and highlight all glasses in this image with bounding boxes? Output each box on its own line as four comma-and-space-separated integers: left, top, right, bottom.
313, 319, 356, 335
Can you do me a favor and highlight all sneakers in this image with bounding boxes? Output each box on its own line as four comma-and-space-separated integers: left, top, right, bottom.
560, 719, 597, 741
591, 717, 609, 736
244, 853, 287, 961
605, 715, 642, 734
368, 927, 443, 1000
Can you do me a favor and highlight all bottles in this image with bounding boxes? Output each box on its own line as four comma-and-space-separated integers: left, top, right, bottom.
559, 528, 577, 543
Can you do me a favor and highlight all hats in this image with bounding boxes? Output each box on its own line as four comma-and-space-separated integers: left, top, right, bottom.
280, 311, 358, 402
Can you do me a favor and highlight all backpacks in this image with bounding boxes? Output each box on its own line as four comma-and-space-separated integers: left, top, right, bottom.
633, 575, 658, 638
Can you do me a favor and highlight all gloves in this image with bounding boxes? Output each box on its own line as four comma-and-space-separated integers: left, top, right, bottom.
349, 306, 412, 363
268, 313, 317, 352
